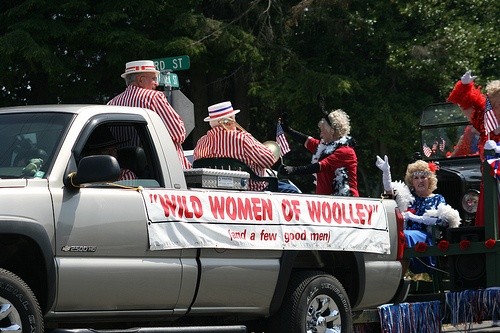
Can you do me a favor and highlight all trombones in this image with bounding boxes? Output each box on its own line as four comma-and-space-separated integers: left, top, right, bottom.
219, 117, 281, 177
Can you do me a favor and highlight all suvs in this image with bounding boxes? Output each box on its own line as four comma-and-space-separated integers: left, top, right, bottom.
404, 101, 500, 302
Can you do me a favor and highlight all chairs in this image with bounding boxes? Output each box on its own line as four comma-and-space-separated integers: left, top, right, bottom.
193, 157, 278, 193
395, 218, 449, 302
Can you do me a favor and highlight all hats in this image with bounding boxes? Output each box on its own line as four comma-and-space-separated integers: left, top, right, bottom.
121, 60, 159, 78
203, 101, 240, 122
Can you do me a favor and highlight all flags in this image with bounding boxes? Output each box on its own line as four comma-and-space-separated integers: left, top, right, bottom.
483, 96, 498, 134
436, 136, 446, 152
276, 123, 290, 157
423, 142, 432, 157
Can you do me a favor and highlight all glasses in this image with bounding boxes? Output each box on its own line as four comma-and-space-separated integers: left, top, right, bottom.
141, 76, 157, 81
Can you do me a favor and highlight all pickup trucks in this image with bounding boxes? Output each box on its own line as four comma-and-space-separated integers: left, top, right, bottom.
0, 104, 412, 333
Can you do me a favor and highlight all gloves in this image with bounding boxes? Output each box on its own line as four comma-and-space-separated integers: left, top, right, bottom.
461, 70, 477, 85
280, 163, 320, 176
484, 140, 497, 150
405, 211, 437, 225
376, 155, 394, 191
280, 112, 308, 144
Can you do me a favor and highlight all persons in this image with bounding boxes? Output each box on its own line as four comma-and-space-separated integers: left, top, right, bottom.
194, 101, 299, 193
375, 155, 461, 274
83, 126, 135, 180
106, 60, 193, 169
278, 109, 359, 196
449, 70, 500, 173
451, 126, 479, 159
